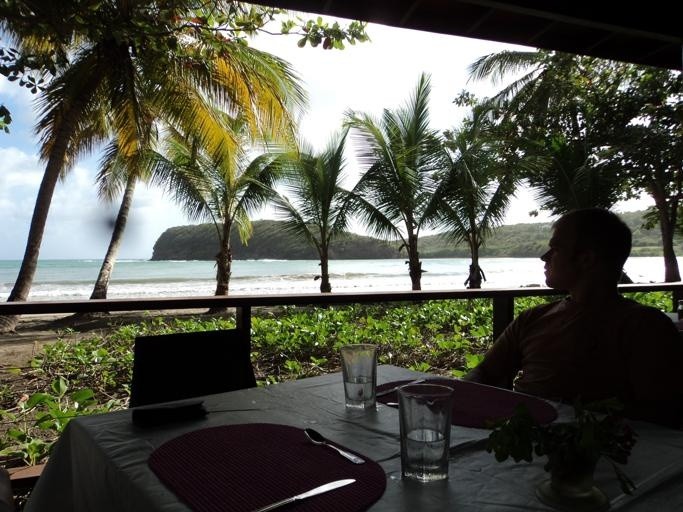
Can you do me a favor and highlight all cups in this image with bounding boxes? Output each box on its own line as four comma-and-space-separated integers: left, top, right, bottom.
397, 383, 455, 482
339, 344, 379, 409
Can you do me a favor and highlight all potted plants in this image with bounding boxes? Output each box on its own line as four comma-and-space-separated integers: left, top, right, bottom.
486, 380, 642, 510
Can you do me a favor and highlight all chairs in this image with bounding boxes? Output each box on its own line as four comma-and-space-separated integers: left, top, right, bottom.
130, 327, 256, 410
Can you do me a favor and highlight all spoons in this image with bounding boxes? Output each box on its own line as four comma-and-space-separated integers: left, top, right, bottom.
304, 427, 366, 465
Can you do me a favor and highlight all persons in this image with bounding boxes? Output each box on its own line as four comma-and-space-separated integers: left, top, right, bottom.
460, 208, 681, 421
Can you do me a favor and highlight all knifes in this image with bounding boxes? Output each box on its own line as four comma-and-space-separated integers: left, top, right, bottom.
255, 478, 356, 511
